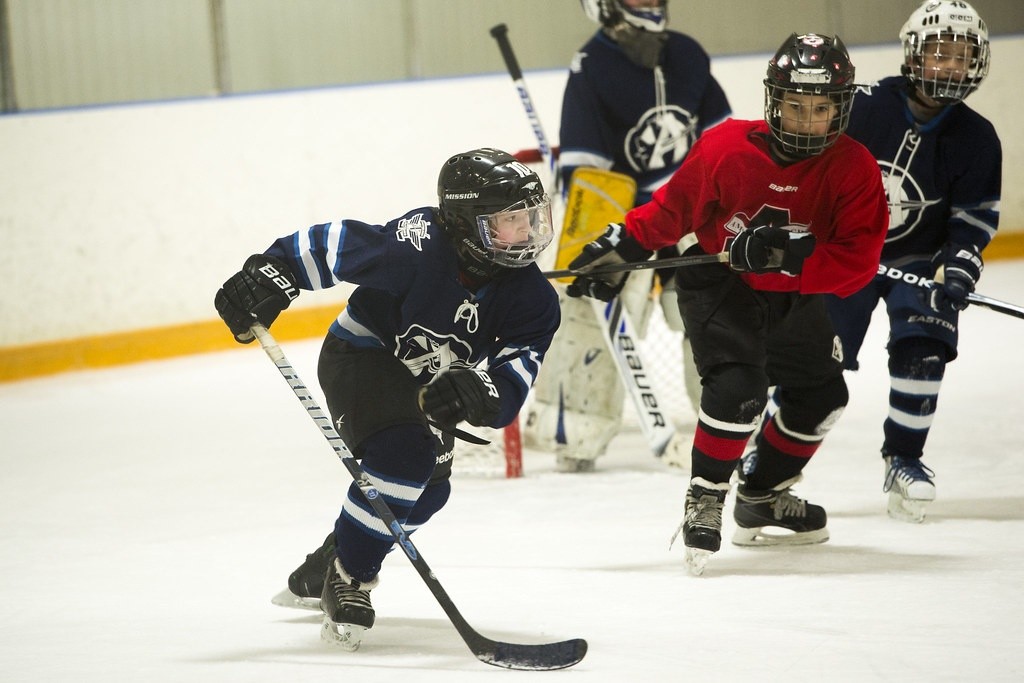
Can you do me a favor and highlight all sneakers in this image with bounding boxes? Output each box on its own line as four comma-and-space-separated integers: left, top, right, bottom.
883, 454, 936, 523
668, 477, 732, 576
741, 448, 758, 476
272, 531, 338, 612
732, 459, 829, 547
319, 531, 380, 652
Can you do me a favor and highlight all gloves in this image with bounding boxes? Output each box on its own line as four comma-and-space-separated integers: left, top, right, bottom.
565, 223, 654, 303
423, 369, 502, 428
729, 226, 816, 278
215, 254, 300, 344
930, 245, 984, 317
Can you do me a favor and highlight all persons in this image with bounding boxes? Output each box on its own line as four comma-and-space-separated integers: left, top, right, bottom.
215, 148, 562, 651
566, 32, 889, 575
753, 0, 1003, 524
527, 0, 734, 472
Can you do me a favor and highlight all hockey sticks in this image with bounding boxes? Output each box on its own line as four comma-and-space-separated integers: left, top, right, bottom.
541, 251, 730, 279
489, 22, 689, 472
250, 318, 588, 671
877, 264, 1024, 320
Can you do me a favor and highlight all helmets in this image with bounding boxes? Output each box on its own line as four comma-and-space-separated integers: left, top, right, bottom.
763, 33, 857, 156
580, 0, 669, 69
899, 0, 991, 106
437, 148, 544, 241
